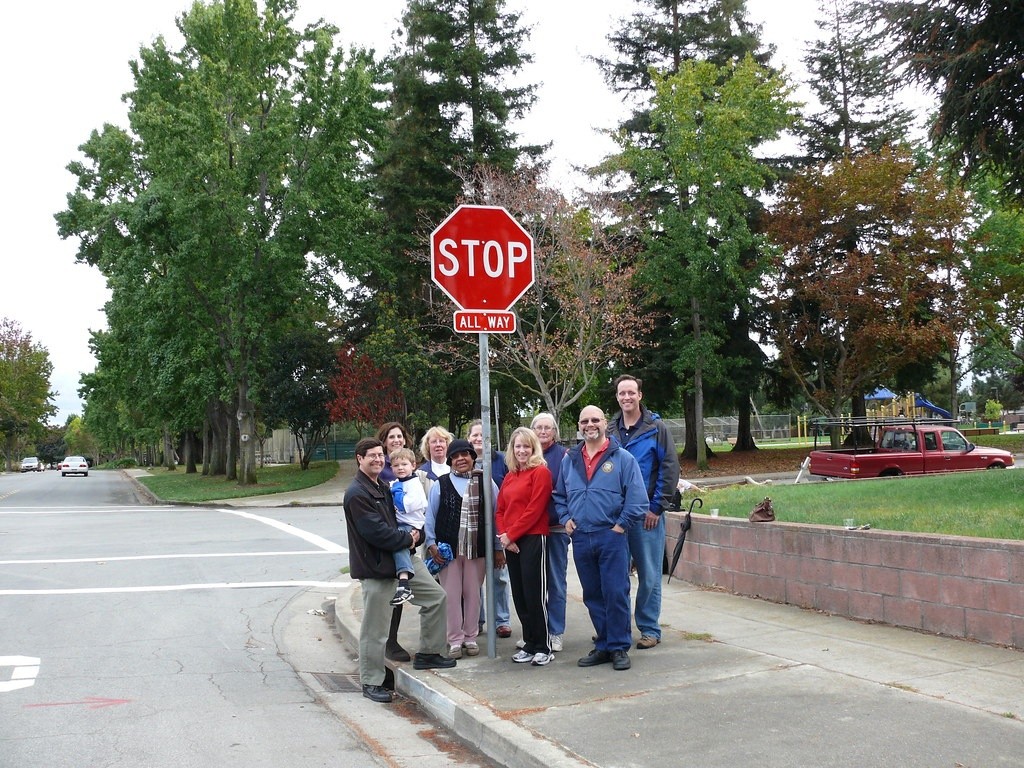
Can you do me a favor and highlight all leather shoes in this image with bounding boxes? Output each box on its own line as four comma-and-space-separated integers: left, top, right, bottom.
413, 653, 456, 669
363, 685, 392, 701
612, 651, 630, 670
578, 649, 612, 666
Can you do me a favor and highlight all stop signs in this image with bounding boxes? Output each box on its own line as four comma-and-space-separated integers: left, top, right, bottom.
429, 203, 536, 312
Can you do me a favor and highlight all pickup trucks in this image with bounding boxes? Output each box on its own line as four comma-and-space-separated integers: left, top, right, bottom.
792, 423, 1015, 484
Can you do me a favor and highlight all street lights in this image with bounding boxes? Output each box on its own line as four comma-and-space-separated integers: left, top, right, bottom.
990, 386, 998, 404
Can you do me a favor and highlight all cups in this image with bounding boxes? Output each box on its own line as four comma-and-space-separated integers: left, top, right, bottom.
843, 519, 853, 529
710, 509, 719, 517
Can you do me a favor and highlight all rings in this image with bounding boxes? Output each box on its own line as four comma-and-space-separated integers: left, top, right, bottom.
501, 545, 504, 548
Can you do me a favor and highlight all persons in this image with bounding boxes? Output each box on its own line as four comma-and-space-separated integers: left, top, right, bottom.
497, 427, 555, 666
390, 448, 427, 605
342, 421, 456, 701
606, 375, 681, 649
517, 413, 569, 652
552, 405, 649, 668
676, 479, 706, 492
424, 440, 499, 658
468, 419, 513, 638
421, 426, 452, 498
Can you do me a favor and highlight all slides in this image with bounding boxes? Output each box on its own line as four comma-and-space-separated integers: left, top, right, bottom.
915, 396, 951, 419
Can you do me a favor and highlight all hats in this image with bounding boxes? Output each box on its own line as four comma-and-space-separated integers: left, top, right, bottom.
446, 439, 477, 466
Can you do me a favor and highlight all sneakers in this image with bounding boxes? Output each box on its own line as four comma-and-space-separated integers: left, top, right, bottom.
511, 650, 534, 662
531, 652, 555, 665
516, 639, 526, 648
549, 633, 563, 652
389, 586, 414, 605
637, 636, 660, 648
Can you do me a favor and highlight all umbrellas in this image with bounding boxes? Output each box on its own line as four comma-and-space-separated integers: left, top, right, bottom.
425, 543, 454, 575
667, 498, 702, 584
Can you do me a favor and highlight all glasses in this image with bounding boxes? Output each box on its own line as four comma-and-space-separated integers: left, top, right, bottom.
449, 451, 468, 459
363, 453, 386, 460
579, 418, 606, 424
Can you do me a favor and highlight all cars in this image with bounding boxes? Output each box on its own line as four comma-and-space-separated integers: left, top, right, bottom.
20, 456, 40, 473
60, 456, 90, 477
40, 461, 62, 471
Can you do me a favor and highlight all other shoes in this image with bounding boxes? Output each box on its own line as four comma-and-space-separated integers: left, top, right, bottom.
386, 639, 411, 661
449, 645, 463, 658
497, 626, 512, 637
465, 642, 479, 656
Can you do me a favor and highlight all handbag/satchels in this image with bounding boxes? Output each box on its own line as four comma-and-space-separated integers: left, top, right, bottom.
749, 497, 775, 522
